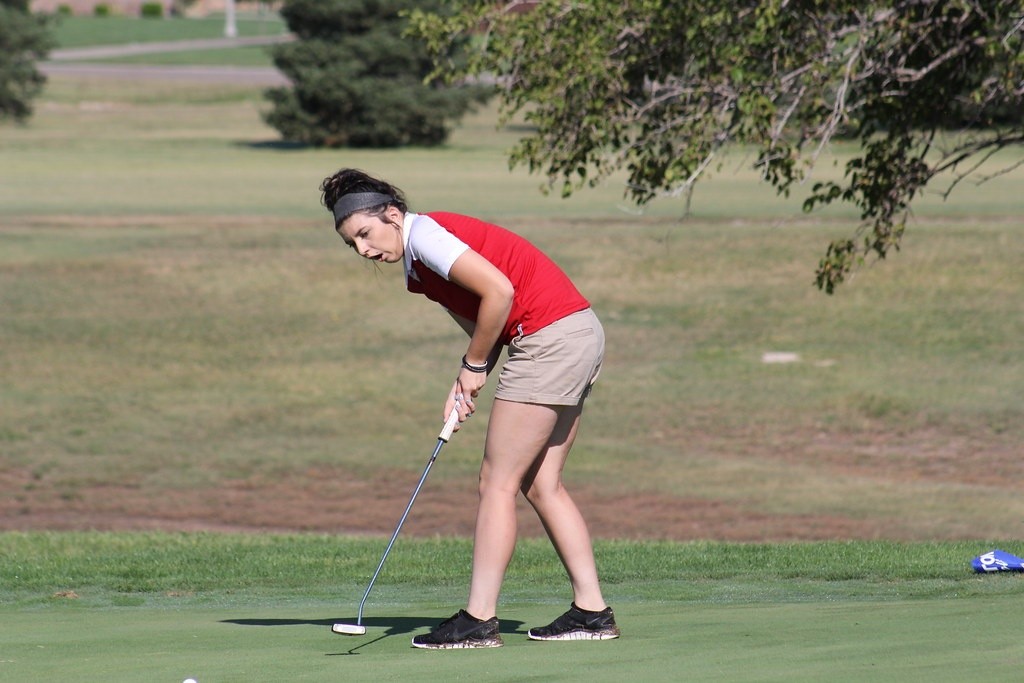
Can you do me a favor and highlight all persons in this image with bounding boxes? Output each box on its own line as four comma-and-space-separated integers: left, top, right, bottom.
321, 169, 624, 653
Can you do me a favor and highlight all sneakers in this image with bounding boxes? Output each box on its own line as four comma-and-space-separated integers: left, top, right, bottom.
412, 603, 619, 650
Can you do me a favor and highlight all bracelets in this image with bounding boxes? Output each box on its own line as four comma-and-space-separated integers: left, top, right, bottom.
461, 354, 490, 375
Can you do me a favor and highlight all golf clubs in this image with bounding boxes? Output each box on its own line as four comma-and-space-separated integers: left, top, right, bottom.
330, 386, 463, 636
